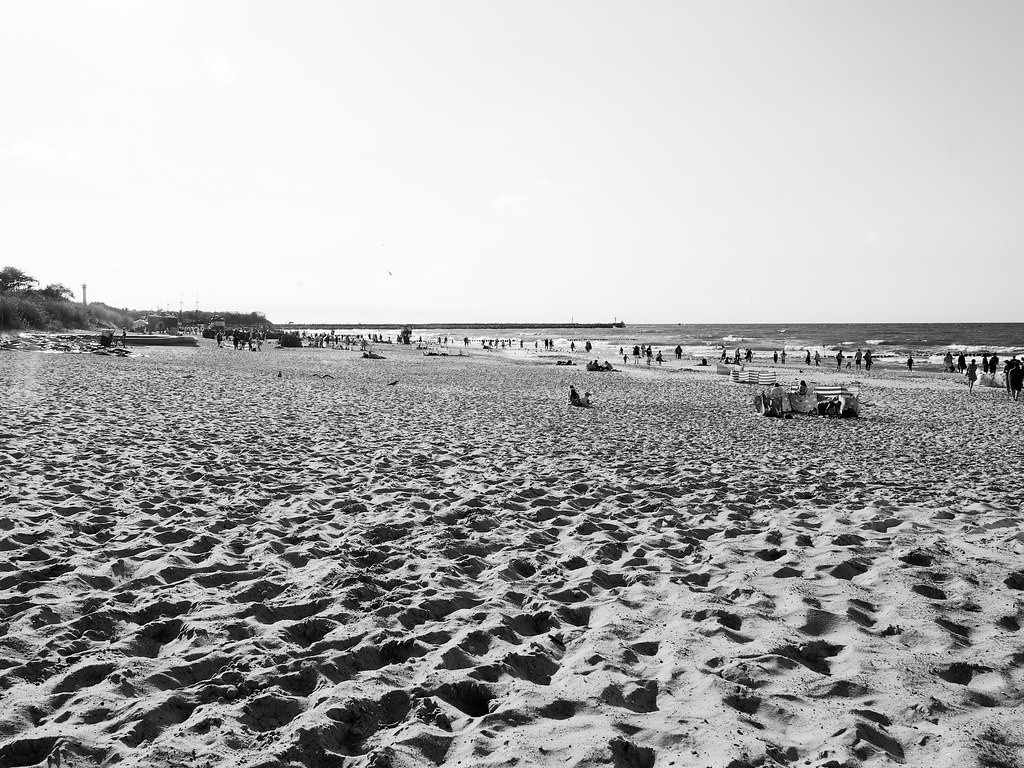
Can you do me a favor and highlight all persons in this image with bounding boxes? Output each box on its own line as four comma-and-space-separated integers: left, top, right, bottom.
140, 322, 596, 354
121, 326, 131, 351
771, 380, 809, 416
718, 344, 875, 370
908, 357, 913, 371
567, 385, 593, 408
619, 342, 707, 367
944, 351, 1024, 403
584, 358, 621, 373
